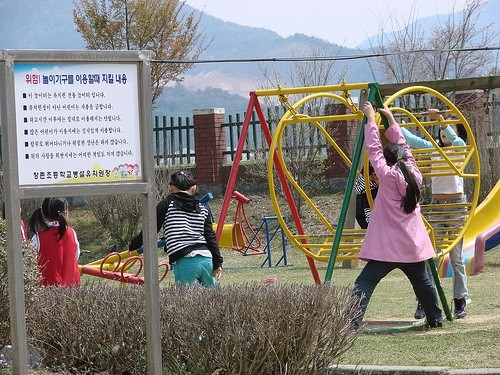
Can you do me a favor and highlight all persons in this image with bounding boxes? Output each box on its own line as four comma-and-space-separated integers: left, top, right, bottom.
131, 171, 223, 288
344, 100, 469, 330
29, 196, 81, 286
2, 202, 27, 253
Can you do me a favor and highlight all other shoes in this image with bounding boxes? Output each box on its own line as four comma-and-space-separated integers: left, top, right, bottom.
428, 321, 443, 327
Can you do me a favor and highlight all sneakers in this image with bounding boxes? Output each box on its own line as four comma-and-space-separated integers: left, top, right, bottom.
451, 297, 467, 318
414, 298, 426, 319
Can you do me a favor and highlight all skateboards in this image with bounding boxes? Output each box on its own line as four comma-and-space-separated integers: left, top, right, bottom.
434, 179, 500, 279
